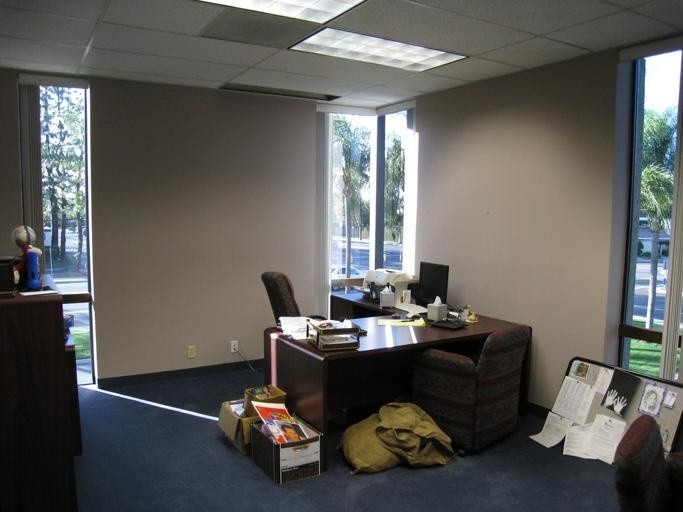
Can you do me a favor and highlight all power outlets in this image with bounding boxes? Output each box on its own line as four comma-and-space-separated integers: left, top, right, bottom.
230, 340, 238, 353
187, 345, 195, 359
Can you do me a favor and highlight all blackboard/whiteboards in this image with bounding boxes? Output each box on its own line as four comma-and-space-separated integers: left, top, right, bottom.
555, 356, 682, 457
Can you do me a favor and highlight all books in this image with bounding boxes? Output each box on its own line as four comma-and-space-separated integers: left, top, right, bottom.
252, 401, 309, 444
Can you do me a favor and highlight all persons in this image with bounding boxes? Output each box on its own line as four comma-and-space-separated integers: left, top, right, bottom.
281, 421, 306, 443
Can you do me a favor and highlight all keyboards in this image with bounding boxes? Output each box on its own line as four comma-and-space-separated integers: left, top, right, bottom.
394, 303, 428, 314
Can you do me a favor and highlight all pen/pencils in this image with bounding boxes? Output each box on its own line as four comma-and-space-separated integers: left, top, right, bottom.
401, 319, 414, 322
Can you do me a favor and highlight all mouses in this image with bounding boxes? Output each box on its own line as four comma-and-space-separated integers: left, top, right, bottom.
405, 313, 420, 318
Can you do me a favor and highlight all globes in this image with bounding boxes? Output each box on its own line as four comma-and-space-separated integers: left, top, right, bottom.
11, 225, 36, 247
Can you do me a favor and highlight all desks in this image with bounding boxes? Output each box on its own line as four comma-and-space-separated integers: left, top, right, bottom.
264, 283, 534, 470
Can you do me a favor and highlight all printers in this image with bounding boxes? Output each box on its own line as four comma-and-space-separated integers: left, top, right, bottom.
353, 269, 411, 294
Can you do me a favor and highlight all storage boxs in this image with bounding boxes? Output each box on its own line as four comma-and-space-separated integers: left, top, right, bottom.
249, 413, 325, 485
243, 384, 287, 416
217, 399, 260, 456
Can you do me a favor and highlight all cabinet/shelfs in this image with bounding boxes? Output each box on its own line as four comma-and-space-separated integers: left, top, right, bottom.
0, 256, 82, 512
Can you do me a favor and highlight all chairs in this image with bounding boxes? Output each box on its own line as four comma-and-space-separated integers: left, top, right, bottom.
412, 328, 531, 455
613, 415, 683, 512
275, 271, 327, 324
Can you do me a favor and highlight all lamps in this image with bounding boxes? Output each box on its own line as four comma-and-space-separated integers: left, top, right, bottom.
196, 0, 372, 25
286, 29, 479, 75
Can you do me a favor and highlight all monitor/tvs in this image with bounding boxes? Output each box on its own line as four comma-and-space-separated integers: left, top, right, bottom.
415, 261, 449, 308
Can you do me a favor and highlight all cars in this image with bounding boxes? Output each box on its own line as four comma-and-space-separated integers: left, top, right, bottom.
331, 265, 363, 291
